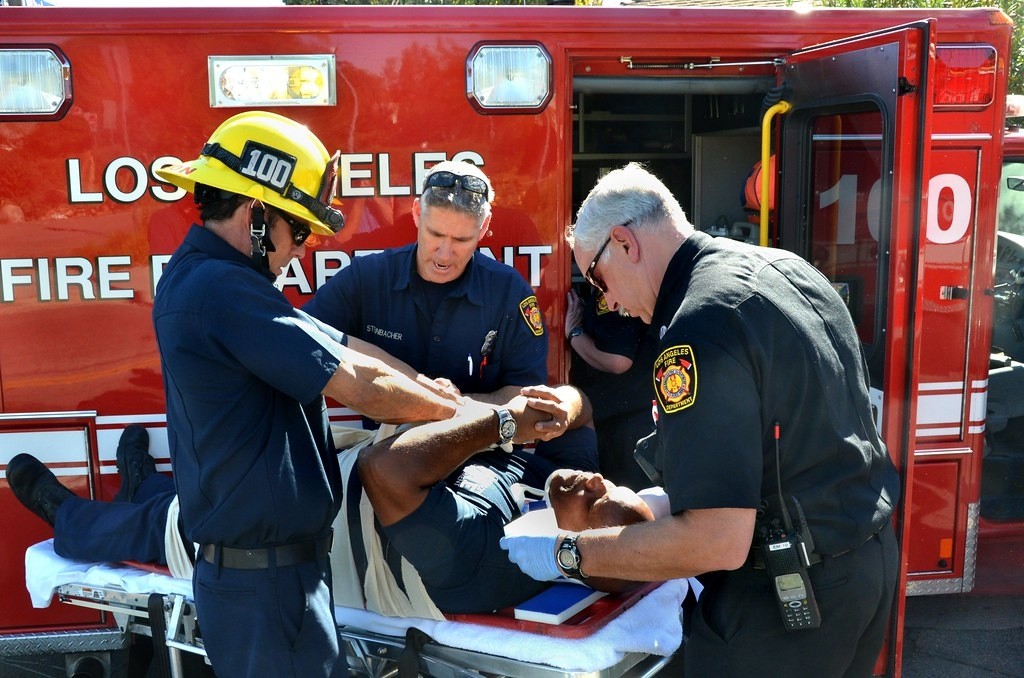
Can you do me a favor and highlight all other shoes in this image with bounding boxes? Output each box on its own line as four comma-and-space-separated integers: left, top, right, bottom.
113, 426, 156, 505
8, 453, 76, 527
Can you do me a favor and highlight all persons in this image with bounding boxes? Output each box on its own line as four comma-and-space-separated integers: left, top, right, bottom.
564, 282, 661, 480
303, 158, 549, 497
151, 107, 510, 677
7, 384, 661, 623
503, 164, 899, 677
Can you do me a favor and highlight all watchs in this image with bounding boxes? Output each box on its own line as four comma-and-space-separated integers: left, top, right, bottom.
492, 405, 517, 445
557, 532, 584, 581
567, 326, 585, 344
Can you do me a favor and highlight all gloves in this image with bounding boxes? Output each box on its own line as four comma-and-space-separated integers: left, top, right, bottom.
637, 485, 671, 519
500, 518, 562, 581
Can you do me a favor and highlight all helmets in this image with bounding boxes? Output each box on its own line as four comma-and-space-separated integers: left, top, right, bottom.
157, 111, 335, 236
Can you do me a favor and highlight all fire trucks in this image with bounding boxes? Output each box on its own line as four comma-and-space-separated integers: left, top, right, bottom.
0, 0, 1024, 656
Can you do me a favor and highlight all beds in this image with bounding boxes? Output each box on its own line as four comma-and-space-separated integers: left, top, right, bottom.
25, 537, 705, 678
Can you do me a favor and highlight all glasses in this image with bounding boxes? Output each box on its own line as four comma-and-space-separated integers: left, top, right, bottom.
422, 171, 488, 201
246, 197, 311, 247
584, 218, 632, 292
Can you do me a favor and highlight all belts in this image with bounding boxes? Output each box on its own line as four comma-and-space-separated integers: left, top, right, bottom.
206, 528, 336, 570
749, 520, 886, 570
